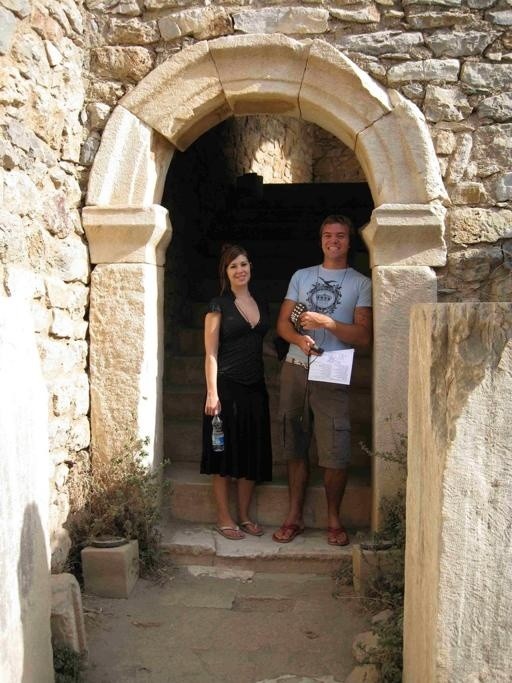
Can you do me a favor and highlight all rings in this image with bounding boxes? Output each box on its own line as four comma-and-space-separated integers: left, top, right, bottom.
207, 410, 211, 413
302, 321, 306, 326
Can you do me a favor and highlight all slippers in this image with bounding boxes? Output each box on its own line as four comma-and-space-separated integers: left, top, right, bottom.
328, 526, 351, 546
237, 519, 264, 536
272, 524, 305, 543
214, 523, 246, 539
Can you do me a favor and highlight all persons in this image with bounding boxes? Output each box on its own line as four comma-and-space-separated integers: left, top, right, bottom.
275, 213, 372, 546
200, 242, 272, 538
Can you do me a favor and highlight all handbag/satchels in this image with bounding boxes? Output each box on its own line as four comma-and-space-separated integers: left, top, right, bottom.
291, 303, 308, 332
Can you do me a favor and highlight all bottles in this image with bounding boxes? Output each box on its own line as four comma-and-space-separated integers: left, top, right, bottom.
211, 414, 225, 452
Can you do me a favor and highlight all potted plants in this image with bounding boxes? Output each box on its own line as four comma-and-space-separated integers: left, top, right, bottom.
61, 412, 175, 600
347, 405, 408, 600
